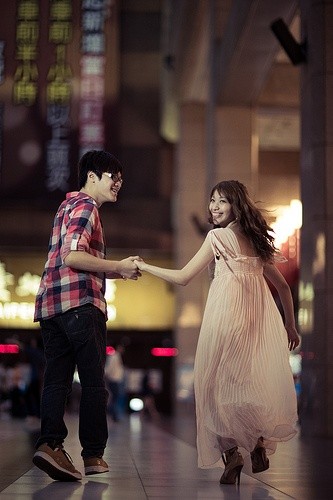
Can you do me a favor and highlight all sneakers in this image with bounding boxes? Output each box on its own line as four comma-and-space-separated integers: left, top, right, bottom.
33, 441, 82, 479
84, 456, 109, 475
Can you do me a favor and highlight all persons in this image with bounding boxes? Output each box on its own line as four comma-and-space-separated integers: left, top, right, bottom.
103, 342, 128, 424
123, 180, 300, 487
32, 150, 143, 482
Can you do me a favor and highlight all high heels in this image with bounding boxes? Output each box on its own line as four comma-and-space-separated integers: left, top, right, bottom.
220, 450, 244, 485
251, 437, 269, 473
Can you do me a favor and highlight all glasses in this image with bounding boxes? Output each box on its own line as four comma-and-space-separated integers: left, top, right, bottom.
99, 172, 123, 184
